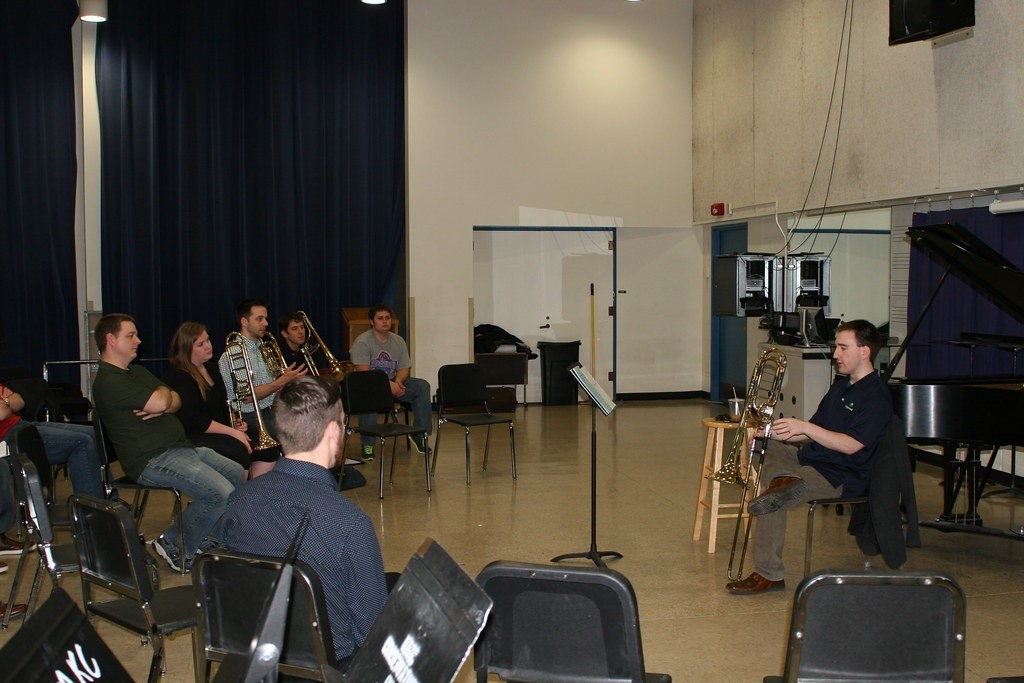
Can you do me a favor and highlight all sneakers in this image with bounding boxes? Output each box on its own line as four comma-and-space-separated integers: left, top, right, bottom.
152, 534, 192, 572
195, 539, 216, 553
408, 433, 433, 454
361, 443, 375, 460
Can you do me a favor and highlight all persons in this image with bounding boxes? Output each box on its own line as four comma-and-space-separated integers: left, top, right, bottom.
218, 301, 308, 459
350, 305, 433, 461
218, 376, 402, 683
93, 314, 246, 572
725, 319, 895, 595
0, 601, 29, 621
0, 458, 39, 574
160, 322, 281, 481
0, 384, 105, 507
278, 312, 319, 377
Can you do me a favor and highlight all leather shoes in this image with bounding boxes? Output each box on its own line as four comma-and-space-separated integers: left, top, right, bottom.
747, 476, 808, 516
0, 600, 28, 619
726, 570, 787, 595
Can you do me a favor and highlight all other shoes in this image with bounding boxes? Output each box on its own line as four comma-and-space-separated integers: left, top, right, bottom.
0, 534, 37, 555
0, 562, 8, 574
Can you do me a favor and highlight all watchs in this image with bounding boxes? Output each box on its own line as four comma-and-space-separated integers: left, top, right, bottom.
2, 397, 10, 408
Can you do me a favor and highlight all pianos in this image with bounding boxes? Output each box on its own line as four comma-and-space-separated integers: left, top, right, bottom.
834, 221, 1024, 542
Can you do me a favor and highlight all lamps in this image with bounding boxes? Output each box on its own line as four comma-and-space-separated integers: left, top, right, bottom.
78, 1, 109, 23
989, 199, 1024, 214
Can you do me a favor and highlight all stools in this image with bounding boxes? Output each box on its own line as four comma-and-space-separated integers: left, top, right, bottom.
692, 417, 752, 553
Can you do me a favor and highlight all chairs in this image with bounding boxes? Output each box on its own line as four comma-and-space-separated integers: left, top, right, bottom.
339, 352, 414, 452
94, 407, 185, 578
66, 492, 196, 683
431, 363, 518, 486
191, 549, 348, 683
762, 571, 966, 683
338, 370, 431, 499
472, 560, 674, 683
1, 453, 158, 628
804, 495, 872, 577
14, 423, 69, 529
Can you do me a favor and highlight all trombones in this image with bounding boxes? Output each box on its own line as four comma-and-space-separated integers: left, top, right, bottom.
703, 347, 787, 582
296, 310, 357, 382
224, 332, 281, 481
